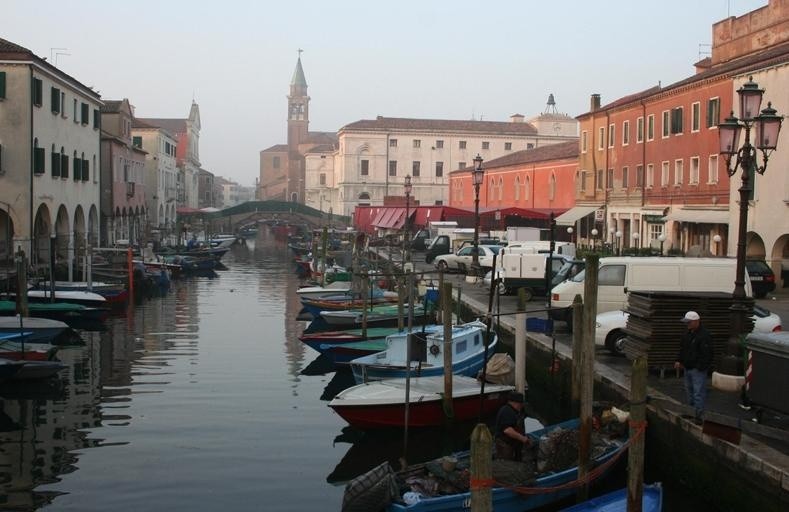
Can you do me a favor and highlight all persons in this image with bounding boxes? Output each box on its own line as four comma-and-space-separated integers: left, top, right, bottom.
492, 390, 534, 464
672, 308, 713, 428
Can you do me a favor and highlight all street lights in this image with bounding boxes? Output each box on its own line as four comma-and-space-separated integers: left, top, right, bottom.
403, 174, 412, 262
719, 74, 783, 378
472, 153, 484, 275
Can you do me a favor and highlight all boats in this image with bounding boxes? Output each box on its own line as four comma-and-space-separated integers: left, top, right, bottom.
1, 227, 258, 380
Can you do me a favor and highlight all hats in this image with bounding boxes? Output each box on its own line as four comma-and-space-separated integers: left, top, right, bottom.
680, 310, 701, 324
504, 391, 528, 405
